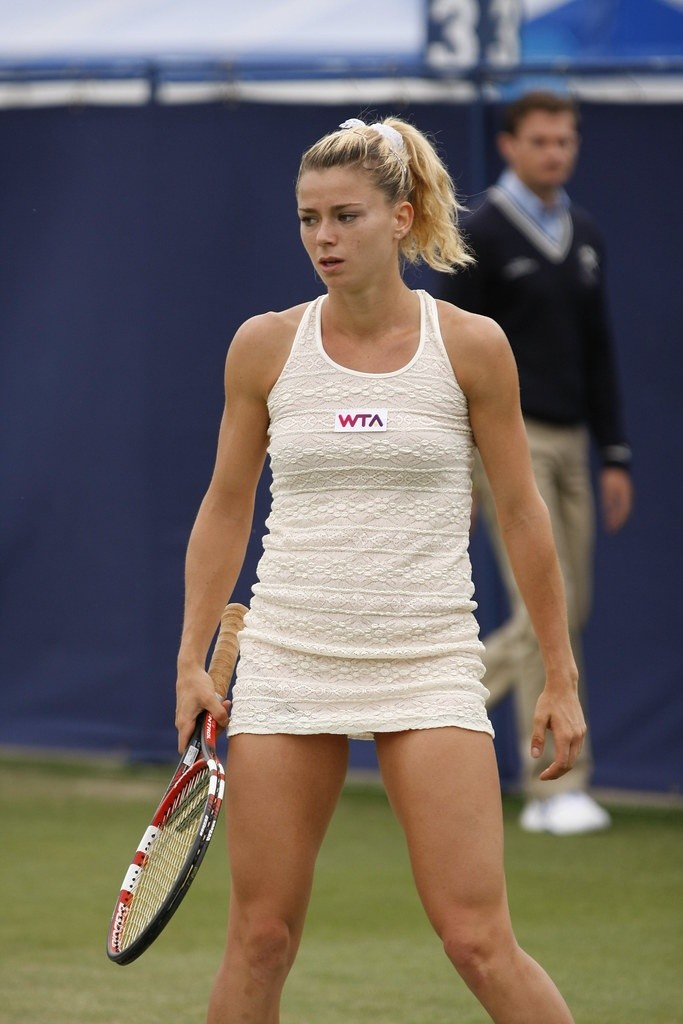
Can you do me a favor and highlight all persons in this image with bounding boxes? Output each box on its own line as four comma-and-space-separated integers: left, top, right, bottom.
173, 119, 587, 1024
431, 92, 638, 840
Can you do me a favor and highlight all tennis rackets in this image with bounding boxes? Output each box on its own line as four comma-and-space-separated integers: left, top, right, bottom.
105, 603, 253, 968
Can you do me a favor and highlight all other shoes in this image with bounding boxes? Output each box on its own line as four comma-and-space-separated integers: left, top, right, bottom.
518, 791, 611, 837
